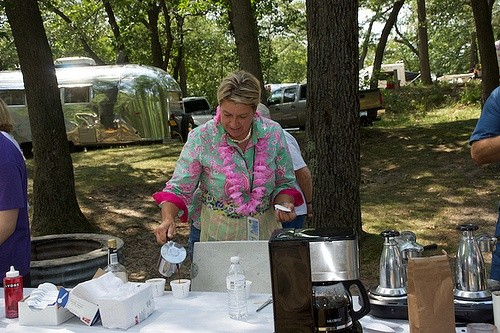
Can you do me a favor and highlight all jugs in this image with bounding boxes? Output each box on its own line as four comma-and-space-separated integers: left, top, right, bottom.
156, 237, 186, 277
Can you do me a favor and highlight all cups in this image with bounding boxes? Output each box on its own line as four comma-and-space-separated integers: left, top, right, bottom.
145, 278, 166, 297
467, 323, 497, 333
492, 291, 500, 330
246, 281, 252, 299
170, 279, 190, 297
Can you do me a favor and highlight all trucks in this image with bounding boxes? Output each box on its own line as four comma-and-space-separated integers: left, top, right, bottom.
0, 56, 184, 154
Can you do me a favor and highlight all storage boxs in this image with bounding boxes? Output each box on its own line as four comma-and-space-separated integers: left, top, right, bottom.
17, 268, 159, 329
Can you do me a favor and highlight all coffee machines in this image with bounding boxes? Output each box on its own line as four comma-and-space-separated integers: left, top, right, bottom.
268, 227, 363, 333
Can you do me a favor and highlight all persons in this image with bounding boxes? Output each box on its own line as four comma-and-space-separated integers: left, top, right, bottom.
151, 70, 304, 265
255, 103, 314, 230
0, 97, 32, 288
469, 86, 500, 282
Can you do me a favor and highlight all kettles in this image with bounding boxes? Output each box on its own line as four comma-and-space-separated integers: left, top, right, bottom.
311, 280, 371, 333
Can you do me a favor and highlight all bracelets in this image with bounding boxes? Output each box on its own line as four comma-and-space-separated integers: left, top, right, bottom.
306, 199, 313, 204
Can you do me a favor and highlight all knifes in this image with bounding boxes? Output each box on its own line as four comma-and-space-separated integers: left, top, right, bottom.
256, 299, 272, 312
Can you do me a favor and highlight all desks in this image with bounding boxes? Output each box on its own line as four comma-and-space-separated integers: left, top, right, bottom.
0, 286, 468, 333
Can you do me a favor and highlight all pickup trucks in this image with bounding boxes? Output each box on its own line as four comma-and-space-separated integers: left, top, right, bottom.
265, 83, 384, 131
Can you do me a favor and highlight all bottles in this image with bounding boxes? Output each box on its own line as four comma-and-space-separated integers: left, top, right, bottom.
103, 239, 128, 284
4, 266, 24, 319
455, 224, 498, 292
226, 256, 249, 320
378, 229, 422, 288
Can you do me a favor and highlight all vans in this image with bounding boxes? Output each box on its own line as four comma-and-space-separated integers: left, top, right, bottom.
359, 61, 406, 90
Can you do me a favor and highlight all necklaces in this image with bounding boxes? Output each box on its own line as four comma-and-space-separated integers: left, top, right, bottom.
228, 124, 252, 144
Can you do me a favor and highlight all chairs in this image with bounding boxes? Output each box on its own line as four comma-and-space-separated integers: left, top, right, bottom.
190, 239, 271, 293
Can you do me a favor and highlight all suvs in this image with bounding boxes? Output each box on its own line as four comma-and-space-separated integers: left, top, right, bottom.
182, 97, 213, 127
